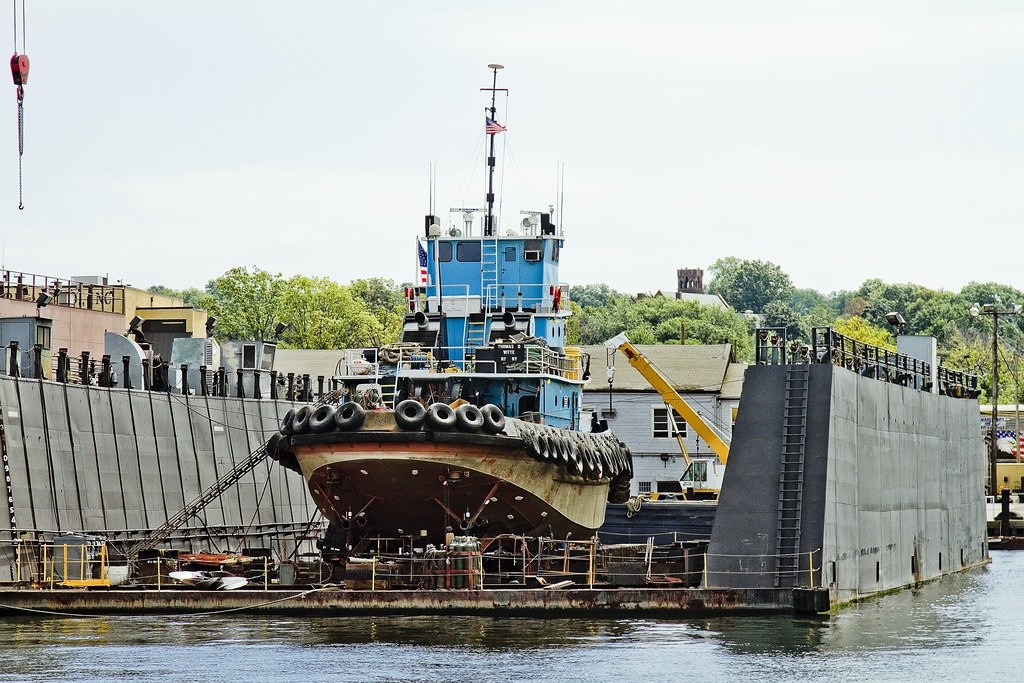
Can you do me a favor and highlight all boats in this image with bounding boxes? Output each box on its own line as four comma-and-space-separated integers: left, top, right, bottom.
260, 60, 634, 576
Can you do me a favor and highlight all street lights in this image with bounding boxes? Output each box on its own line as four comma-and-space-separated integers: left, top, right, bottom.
968, 301, 1023, 499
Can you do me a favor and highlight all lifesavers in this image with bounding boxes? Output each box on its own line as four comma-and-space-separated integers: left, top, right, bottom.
409, 288, 414, 312
555, 286, 561, 311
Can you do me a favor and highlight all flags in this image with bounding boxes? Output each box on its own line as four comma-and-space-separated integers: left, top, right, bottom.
996, 429, 1024, 459
417, 240, 428, 283
485, 116, 507, 135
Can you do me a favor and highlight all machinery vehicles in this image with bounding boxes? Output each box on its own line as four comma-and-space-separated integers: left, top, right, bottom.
602, 331, 738, 501
